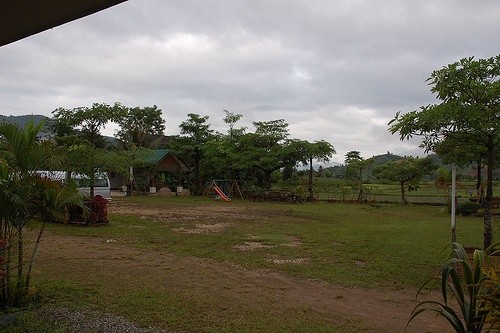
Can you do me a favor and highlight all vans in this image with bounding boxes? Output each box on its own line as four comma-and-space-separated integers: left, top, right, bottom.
17, 171, 112, 202
